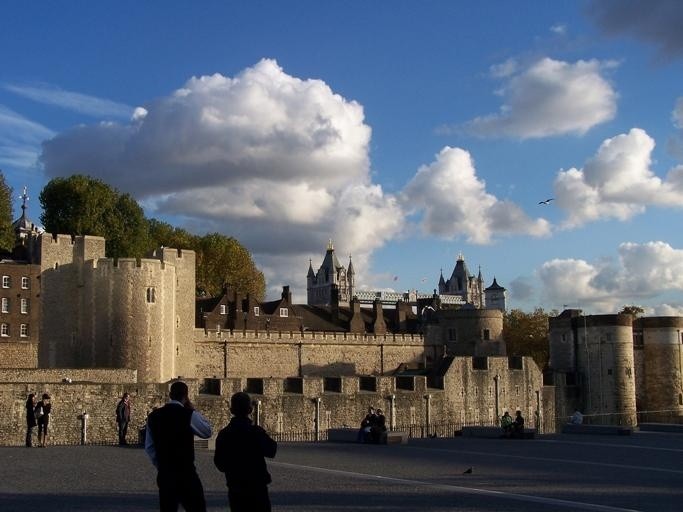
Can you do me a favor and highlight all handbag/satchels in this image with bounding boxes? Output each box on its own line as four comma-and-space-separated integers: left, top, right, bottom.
34, 401, 44, 419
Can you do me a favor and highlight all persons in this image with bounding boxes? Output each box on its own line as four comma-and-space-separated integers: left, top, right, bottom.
33, 393, 51, 449
357, 406, 377, 444
514, 410, 525, 432
25, 393, 36, 448
142, 381, 212, 512
500, 412, 512, 428
214, 391, 279, 512
114, 392, 132, 445
369, 408, 387, 444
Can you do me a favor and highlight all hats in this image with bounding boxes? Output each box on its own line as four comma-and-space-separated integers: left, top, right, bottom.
42, 394, 51, 400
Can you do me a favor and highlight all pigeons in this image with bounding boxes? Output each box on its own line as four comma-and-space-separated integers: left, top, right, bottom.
463, 467, 473, 474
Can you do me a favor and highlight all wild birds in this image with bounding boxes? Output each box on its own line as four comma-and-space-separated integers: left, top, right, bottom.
537, 199, 553, 206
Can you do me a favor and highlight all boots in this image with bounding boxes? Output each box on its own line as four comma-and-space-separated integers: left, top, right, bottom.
37, 435, 42, 447
43, 434, 48, 449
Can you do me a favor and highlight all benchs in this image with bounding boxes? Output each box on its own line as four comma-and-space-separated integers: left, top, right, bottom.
328, 428, 404, 445
454, 426, 536, 439
565, 423, 633, 436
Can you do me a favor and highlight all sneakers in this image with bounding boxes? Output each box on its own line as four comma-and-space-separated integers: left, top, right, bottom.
26, 444, 37, 448
